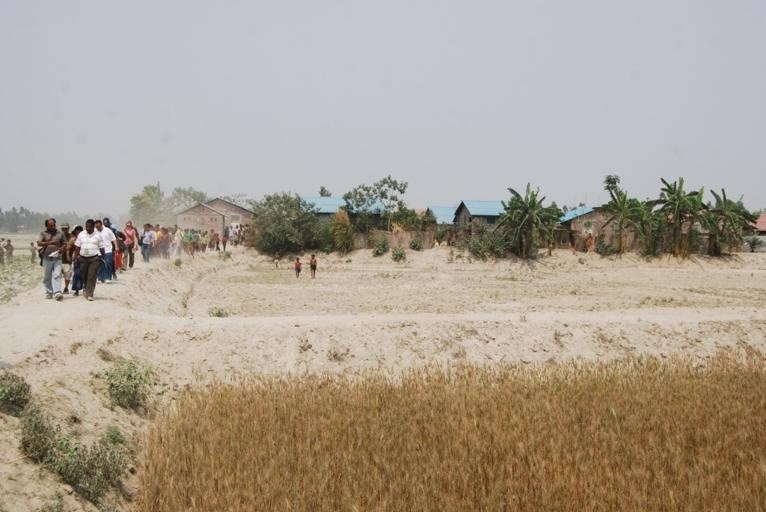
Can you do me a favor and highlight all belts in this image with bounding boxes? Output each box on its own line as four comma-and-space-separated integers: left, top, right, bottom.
79, 254, 98, 259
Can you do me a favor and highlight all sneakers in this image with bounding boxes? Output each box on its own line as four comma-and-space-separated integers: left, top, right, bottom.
44, 289, 95, 301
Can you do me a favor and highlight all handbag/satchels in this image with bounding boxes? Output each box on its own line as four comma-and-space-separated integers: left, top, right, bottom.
38, 247, 45, 266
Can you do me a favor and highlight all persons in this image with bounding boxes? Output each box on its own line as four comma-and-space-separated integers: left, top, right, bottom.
28, 241, 37, 264
306, 254, 317, 279
294, 256, 301, 278
3, 239, 14, 264
34, 217, 250, 301
0, 238, 5, 265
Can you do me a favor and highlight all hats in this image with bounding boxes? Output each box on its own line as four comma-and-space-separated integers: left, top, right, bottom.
125, 222, 131, 226
